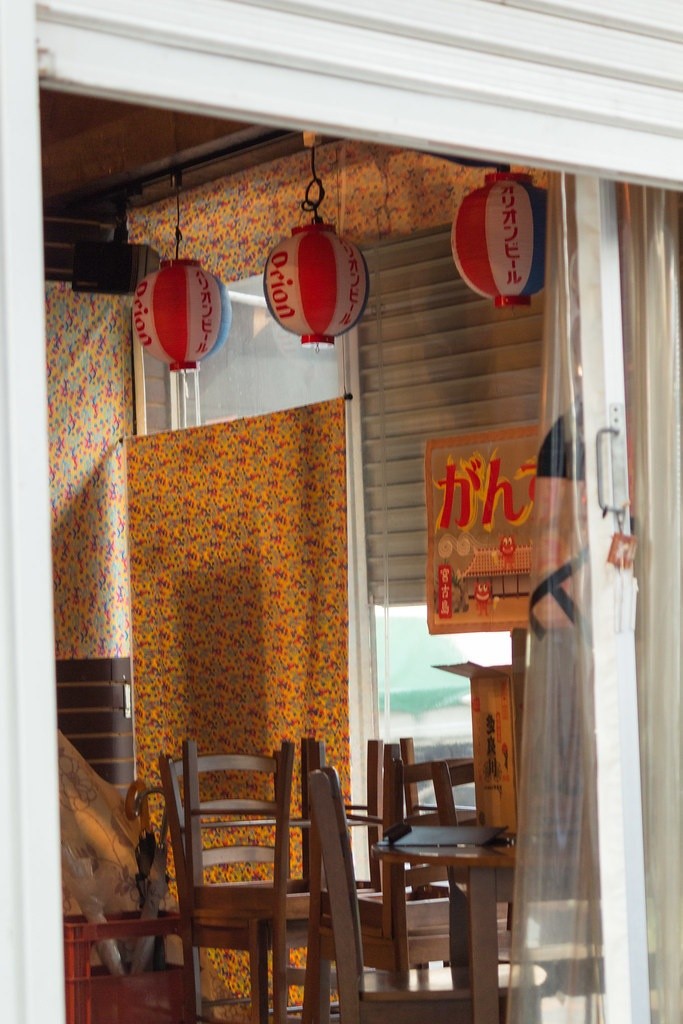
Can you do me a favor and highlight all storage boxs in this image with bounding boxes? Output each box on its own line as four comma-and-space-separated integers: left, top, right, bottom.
434, 660, 526, 834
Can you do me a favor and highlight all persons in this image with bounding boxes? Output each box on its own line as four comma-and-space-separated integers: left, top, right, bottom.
529, 413, 585, 628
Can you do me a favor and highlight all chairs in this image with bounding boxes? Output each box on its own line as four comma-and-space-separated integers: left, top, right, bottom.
156, 730, 513, 1024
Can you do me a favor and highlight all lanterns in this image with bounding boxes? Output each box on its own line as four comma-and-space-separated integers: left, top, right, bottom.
263, 223, 369, 348
451, 172, 548, 310
132, 258, 232, 372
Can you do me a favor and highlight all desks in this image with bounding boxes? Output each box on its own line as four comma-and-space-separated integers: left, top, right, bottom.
371, 830, 596, 1024
